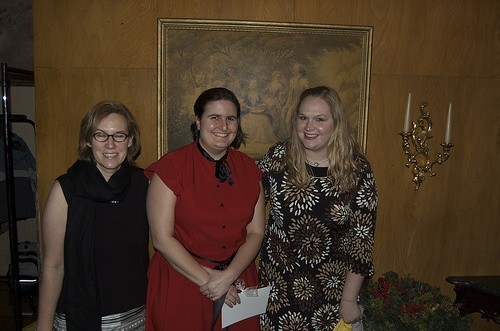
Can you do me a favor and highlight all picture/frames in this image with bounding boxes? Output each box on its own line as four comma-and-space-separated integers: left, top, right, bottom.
157, 17, 374, 161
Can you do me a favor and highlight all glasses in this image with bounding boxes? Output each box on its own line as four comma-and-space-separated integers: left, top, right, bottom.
93, 132, 131, 142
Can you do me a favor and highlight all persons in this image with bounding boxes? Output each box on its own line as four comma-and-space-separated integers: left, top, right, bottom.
36, 100, 151, 331
144, 87, 267, 330
254, 86, 380, 331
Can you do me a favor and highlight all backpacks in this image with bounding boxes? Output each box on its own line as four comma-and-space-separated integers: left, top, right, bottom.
8, 240, 40, 286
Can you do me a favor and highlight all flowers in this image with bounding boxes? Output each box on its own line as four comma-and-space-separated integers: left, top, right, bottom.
357, 270, 471, 331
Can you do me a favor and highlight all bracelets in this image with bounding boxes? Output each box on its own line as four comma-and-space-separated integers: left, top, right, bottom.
341, 295, 360, 303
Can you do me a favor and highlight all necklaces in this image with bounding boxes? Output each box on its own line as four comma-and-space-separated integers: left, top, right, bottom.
304, 156, 329, 167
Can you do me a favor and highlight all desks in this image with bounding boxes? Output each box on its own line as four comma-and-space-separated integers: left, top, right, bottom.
445, 276, 500, 322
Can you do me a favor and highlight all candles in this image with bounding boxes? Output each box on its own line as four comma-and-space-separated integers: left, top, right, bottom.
404, 92, 411, 131
444, 103, 452, 144
